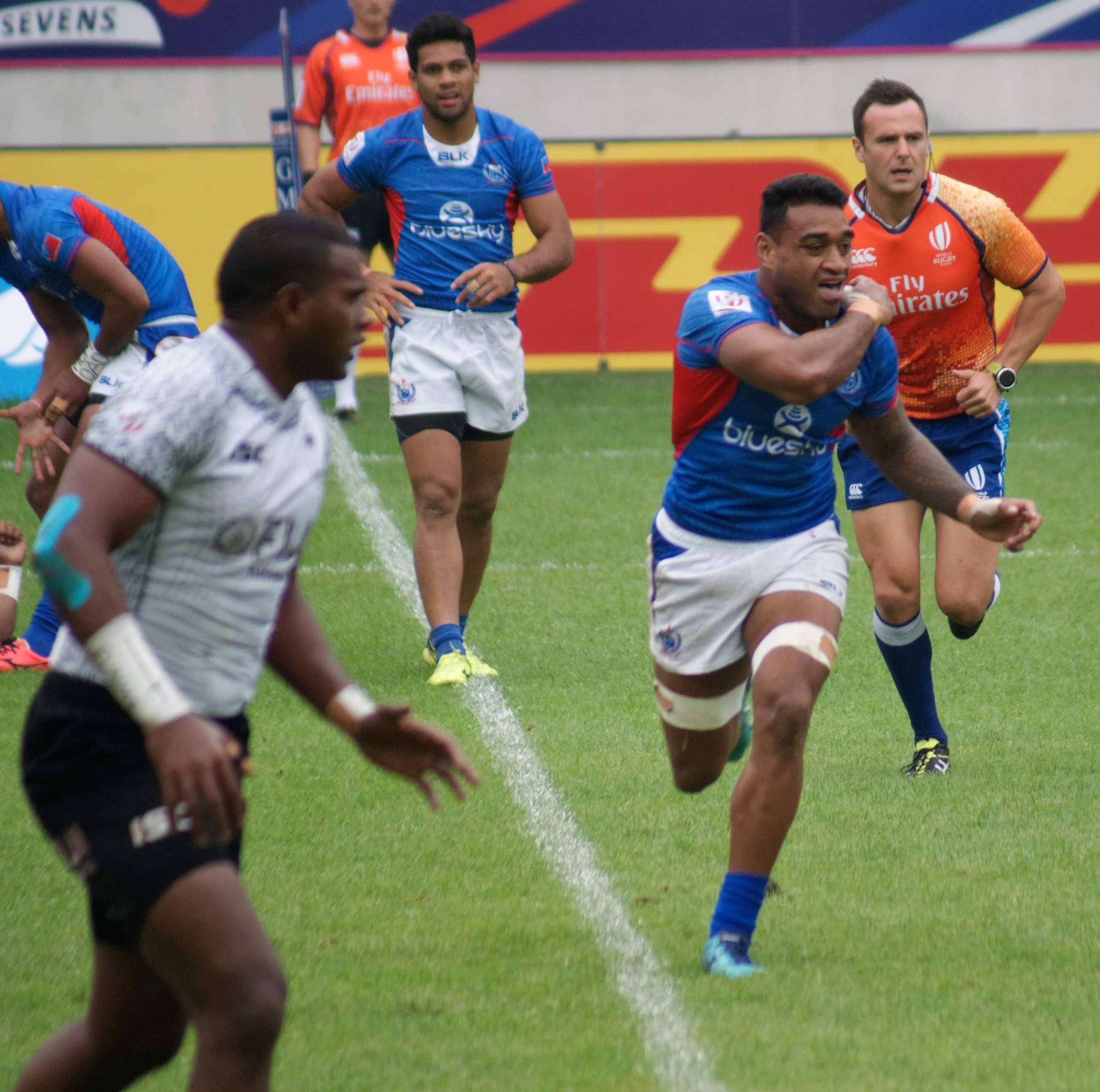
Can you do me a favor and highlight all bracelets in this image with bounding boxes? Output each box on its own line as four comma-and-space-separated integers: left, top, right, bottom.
302, 168, 316, 188
327, 682, 378, 733
29, 398, 44, 411
846, 299, 883, 329
362, 268, 372, 279
502, 260, 518, 284
956, 494, 979, 525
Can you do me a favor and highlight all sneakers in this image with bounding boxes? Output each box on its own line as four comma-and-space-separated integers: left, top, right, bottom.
1, 638, 50, 675
425, 650, 470, 686
423, 645, 499, 681
899, 739, 952, 782
725, 678, 753, 763
701, 930, 763, 980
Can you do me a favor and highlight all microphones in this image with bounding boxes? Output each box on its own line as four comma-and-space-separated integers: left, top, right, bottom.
920, 153, 937, 189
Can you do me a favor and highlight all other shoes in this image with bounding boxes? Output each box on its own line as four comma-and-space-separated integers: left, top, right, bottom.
334, 390, 358, 422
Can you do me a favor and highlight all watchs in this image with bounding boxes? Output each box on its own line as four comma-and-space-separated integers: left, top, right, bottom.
985, 362, 1018, 393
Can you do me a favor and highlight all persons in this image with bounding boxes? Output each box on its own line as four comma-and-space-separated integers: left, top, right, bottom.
299, 14, 576, 684
834, 80, 1065, 776
15, 213, 484, 1092
0, 181, 202, 674
648, 172, 1041, 989
289, 0, 424, 417
